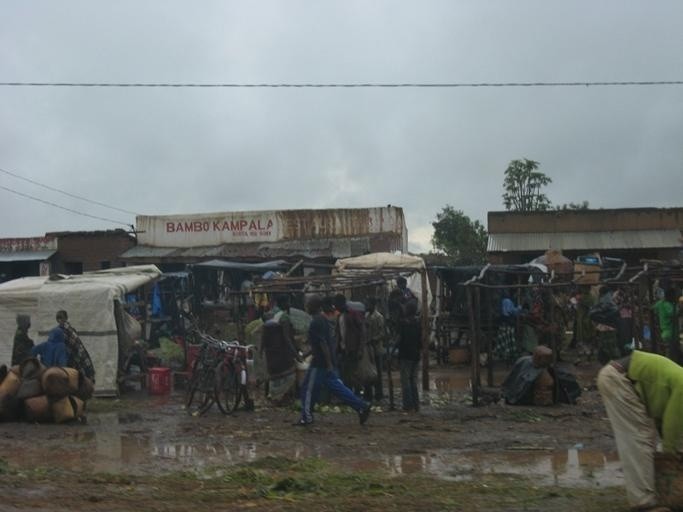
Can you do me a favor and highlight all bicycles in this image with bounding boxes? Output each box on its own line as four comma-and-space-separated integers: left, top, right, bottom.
185, 324, 254, 417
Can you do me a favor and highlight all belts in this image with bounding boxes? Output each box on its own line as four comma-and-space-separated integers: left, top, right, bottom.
609, 359, 625, 373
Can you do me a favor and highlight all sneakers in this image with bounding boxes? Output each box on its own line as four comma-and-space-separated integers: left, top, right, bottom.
294, 418, 314, 425
358, 401, 371, 423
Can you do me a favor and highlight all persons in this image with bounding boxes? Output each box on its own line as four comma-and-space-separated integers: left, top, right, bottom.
598, 349, 683, 512
11, 310, 95, 385
262, 277, 425, 425
497, 279, 683, 366
